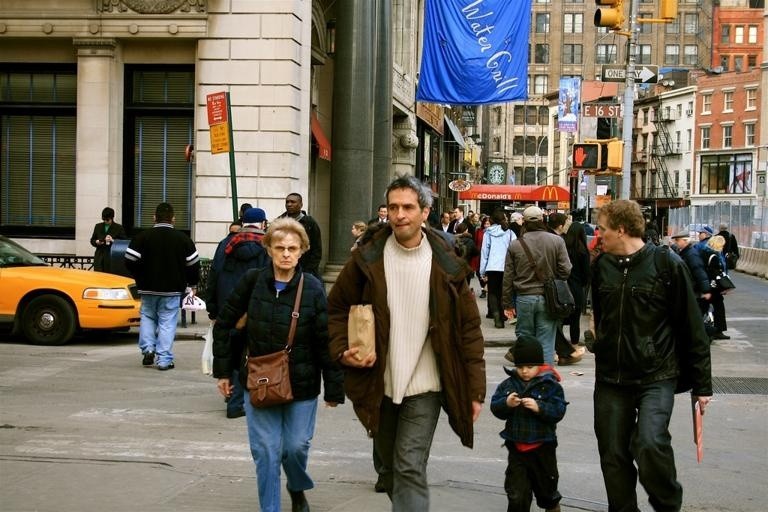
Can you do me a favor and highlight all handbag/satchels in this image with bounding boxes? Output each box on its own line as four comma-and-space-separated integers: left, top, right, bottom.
246, 348, 296, 408
544, 279, 577, 321
714, 276, 736, 295
725, 252, 737, 270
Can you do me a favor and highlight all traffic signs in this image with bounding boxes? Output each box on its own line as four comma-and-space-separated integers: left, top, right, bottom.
600, 64, 660, 84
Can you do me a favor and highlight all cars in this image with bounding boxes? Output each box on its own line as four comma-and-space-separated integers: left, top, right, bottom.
750, 230, 768, 249
0, 228, 143, 346
683, 223, 709, 243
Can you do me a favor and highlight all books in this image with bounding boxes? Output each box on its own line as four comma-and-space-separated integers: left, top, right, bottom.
692, 400, 703, 464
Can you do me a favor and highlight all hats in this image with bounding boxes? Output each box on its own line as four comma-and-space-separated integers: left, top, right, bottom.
513, 335, 546, 365
242, 208, 268, 223
696, 226, 713, 235
523, 206, 543, 222
671, 230, 690, 239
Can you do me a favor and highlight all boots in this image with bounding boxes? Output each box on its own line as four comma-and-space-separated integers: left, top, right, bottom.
493, 311, 505, 329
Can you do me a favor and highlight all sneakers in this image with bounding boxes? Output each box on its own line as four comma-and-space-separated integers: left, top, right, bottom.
479, 290, 487, 298
158, 361, 176, 371
505, 349, 514, 363
714, 332, 730, 340
375, 476, 387, 493
226, 410, 246, 418
289, 491, 310, 511
142, 350, 155, 365
557, 355, 583, 366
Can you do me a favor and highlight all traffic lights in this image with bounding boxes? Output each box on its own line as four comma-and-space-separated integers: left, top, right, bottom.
593, 0, 625, 30
582, 103, 621, 119
568, 141, 604, 173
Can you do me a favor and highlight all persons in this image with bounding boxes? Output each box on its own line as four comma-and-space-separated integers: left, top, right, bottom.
348, 201, 743, 371
123, 202, 203, 372
90, 206, 129, 275
238, 204, 252, 223
587, 201, 714, 511
489, 334, 571, 511
205, 207, 273, 419
327, 171, 488, 512
214, 221, 242, 256
211, 216, 346, 511
276, 192, 322, 275
